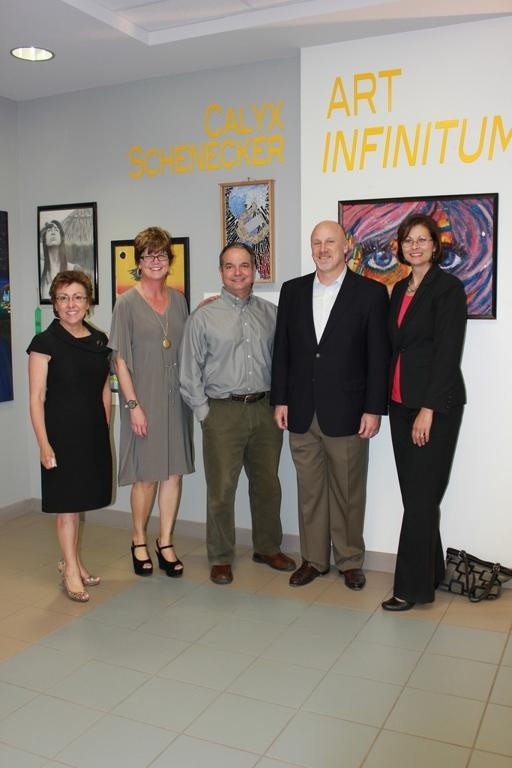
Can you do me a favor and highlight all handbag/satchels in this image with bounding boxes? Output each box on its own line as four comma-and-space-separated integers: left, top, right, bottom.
437, 548, 511, 602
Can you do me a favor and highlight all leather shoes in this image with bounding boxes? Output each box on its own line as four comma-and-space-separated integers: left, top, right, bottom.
382, 597, 415, 611
210, 565, 232, 584
252, 552, 296, 571
339, 569, 366, 591
400, 234, 434, 249
288, 560, 330, 588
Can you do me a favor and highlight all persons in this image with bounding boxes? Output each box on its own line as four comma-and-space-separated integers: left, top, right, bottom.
106, 226, 195, 577
40, 220, 79, 300
271, 221, 390, 590
382, 213, 467, 611
178, 243, 296, 584
26, 270, 113, 603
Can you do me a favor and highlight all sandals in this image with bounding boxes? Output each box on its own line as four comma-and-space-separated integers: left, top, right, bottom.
56, 559, 100, 603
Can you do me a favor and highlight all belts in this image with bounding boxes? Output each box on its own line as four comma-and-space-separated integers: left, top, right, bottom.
227, 392, 265, 405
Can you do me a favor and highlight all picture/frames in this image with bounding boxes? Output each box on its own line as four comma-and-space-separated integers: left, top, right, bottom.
218, 178, 275, 284
336, 191, 498, 322
36, 200, 101, 307
111, 236, 190, 314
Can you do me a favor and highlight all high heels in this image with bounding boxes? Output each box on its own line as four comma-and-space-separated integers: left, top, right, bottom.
131, 541, 153, 575
155, 538, 184, 577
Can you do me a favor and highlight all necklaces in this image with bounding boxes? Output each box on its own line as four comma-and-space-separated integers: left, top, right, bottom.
141, 285, 171, 349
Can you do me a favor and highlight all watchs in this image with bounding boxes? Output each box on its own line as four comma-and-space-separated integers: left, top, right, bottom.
125, 399, 138, 408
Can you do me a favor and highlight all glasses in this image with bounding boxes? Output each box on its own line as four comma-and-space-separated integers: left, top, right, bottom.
139, 255, 170, 263
54, 294, 88, 305
